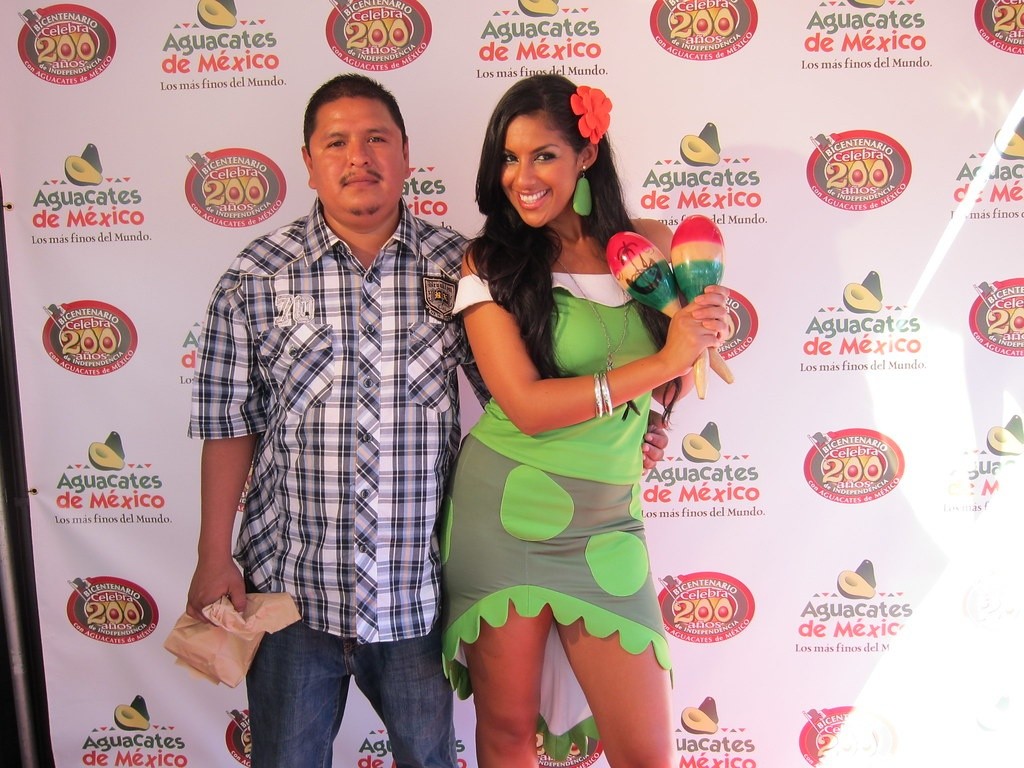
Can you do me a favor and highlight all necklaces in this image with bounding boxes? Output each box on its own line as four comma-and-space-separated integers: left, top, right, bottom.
556, 257, 628, 372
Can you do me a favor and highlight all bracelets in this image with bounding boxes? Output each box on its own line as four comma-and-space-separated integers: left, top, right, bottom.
592, 371, 612, 419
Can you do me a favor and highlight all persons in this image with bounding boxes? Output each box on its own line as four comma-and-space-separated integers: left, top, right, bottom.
185, 73, 668, 768
438, 74, 732, 768
